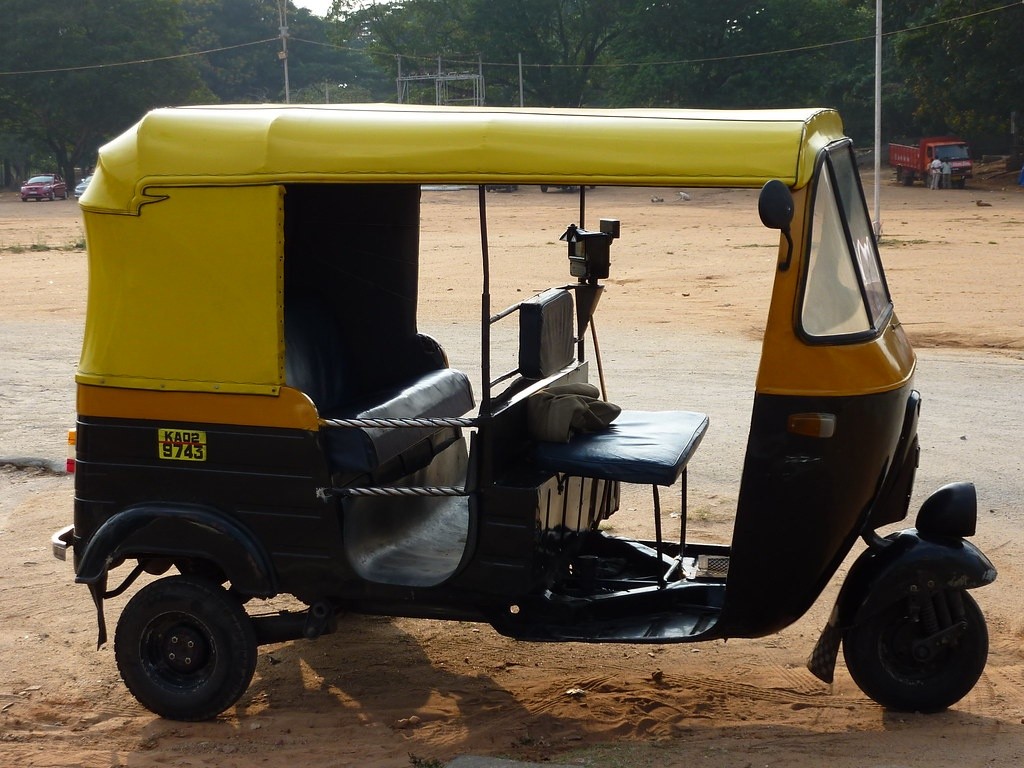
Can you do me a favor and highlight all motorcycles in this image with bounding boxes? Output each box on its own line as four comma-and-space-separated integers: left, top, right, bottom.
51, 103, 998, 719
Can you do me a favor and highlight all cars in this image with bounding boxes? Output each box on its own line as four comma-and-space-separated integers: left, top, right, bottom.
75, 176, 92, 200
20, 174, 68, 201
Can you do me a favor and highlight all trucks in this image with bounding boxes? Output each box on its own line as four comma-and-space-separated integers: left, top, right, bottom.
888, 136, 973, 188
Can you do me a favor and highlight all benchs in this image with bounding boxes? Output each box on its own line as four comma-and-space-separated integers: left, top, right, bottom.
276, 298, 474, 483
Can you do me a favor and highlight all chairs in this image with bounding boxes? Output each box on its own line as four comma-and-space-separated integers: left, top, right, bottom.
520, 289, 709, 586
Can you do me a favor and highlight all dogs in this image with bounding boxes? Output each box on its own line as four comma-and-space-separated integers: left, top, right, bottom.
977, 199, 992, 207
649, 196, 664, 204
673, 191, 691, 202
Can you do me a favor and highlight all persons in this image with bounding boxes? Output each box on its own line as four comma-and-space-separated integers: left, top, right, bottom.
927, 155, 952, 190
